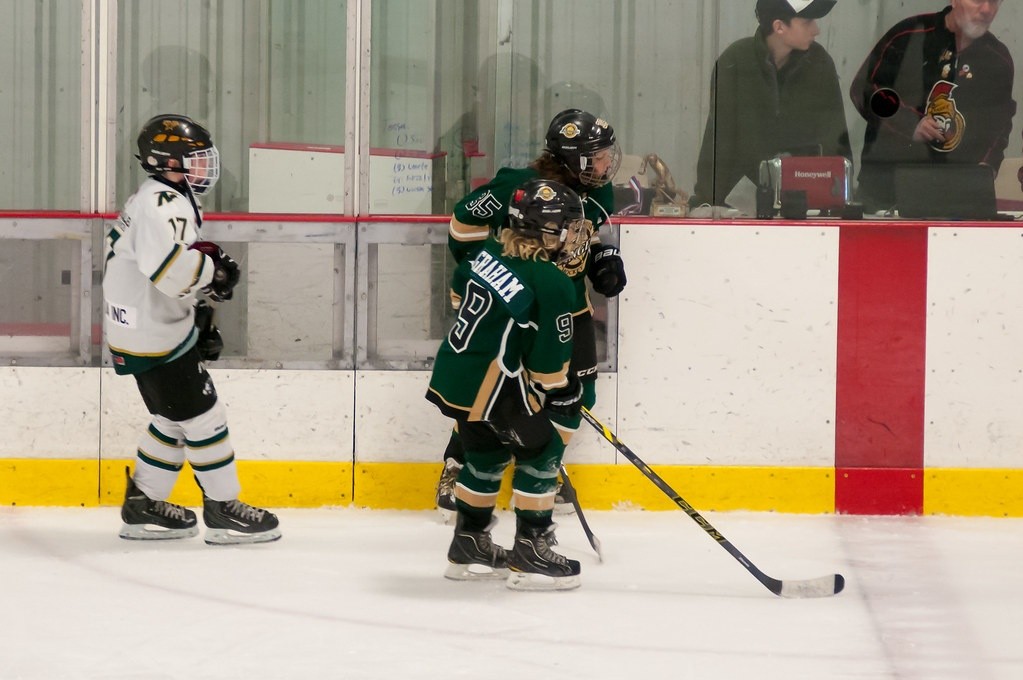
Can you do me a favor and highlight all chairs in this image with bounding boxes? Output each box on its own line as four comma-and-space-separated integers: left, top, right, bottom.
772, 157, 854, 217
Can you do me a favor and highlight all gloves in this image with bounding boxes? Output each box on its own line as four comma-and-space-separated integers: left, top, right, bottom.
586, 246, 627, 297
188, 243, 239, 302
193, 300, 224, 363
545, 373, 584, 417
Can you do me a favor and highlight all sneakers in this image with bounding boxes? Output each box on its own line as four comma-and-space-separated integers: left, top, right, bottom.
193, 474, 281, 545
119, 466, 199, 539
436, 458, 461, 526
507, 518, 582, 590
444, 510, 514, 581
508, 479, 579, 513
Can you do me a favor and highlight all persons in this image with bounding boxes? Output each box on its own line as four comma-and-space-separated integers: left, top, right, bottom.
426, 52, 672, 218
849, 0, 1017, 221
103, 115, 284, 544
423, 110, 627, 590
689, 0, 853, 211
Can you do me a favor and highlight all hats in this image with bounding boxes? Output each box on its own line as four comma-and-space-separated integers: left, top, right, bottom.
756, 0, 838, 28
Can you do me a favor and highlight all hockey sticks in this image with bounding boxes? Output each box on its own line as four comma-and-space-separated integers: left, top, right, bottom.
579, 405, 845, 599
559, 460, 603, 563
203, 292, 216, 334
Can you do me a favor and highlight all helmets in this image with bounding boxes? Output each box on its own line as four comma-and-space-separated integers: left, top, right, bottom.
537, 82, 611, 123
507, 180, 585, 236
479, 51, 545, 107
546, 108, 623, 188
137, 114, 220, 197
